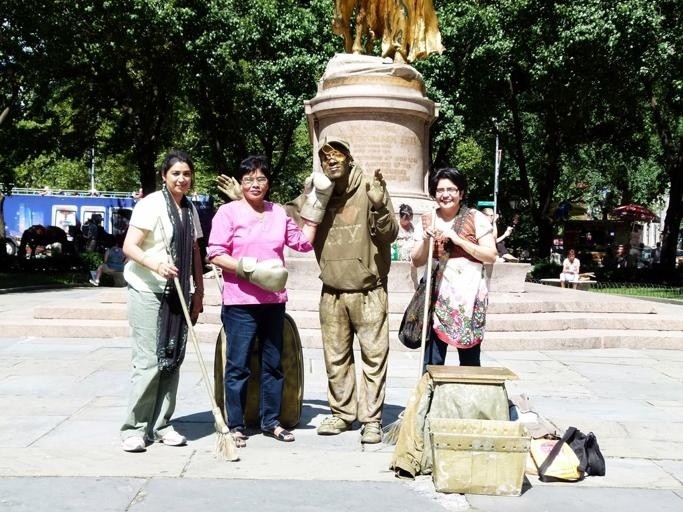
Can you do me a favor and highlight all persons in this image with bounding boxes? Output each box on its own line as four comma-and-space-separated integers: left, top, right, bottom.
117, 151, 204, 452
391, 204, 683, 290
216, 137, 399, 443
24, 214, 124, 286
205, 154, 336, 448
411, 167, 498, 376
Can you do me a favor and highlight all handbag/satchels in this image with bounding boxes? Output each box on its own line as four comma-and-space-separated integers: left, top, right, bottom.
565, 427, 605, 476
399, 278, 435, 348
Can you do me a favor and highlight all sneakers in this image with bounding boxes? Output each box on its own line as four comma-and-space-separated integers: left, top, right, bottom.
361, 422, 381, 443
121, 436, 145, 451
148, 431, 186, 446
317, 417, 352, 435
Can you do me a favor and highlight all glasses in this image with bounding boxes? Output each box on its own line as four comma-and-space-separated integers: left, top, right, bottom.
322, 136, 346, 162
437, 188, 459, 194
240, 175, 268, 185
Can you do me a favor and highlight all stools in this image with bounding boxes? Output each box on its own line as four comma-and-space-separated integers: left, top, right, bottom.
110, 271, 127, 287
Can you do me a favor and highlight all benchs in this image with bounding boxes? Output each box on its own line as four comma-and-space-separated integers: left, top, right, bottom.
47, 266, 83, 283
539, 278, 597, 291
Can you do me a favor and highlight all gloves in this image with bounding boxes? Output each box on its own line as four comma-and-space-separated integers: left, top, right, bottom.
236, 257, 288, 291
300, 172, 335, 224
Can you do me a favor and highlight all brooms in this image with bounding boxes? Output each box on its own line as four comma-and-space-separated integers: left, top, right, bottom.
155, 217, 240, 463
381, 210, 437, 445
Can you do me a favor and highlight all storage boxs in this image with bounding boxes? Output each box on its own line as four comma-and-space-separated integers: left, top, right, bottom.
426, 416, 532, 495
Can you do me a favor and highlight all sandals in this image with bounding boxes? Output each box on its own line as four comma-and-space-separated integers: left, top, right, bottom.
263, 424, 295, 442
232, 431, 248, 447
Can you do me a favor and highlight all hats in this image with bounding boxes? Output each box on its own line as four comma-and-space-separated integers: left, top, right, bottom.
319, 136, 350, 157
